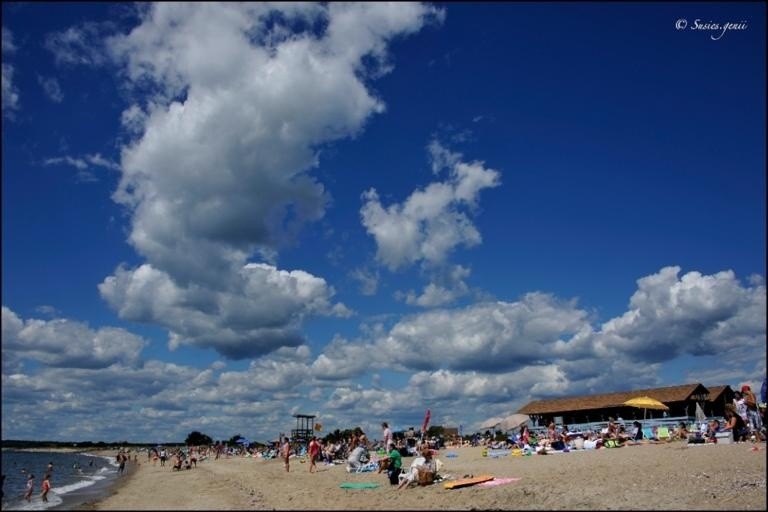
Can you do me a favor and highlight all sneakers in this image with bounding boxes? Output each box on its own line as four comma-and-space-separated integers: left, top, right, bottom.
751, 446, 760, 451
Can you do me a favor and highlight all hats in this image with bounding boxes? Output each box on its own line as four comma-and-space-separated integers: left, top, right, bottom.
740, 386, 750, 394
422, 451, 432, 456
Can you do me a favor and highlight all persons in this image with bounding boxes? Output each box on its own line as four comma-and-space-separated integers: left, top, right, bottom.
40, 472, 52, 503
23, 473, 34, 503
